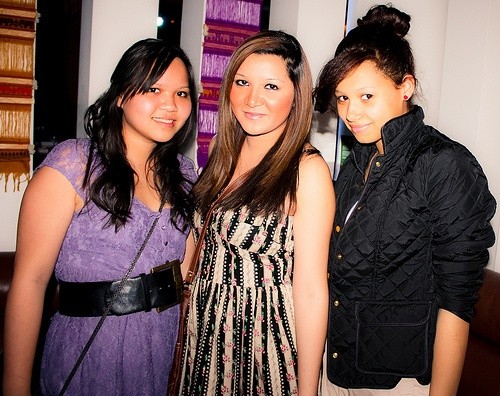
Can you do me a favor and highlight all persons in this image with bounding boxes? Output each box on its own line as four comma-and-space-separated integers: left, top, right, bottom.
316, 4, 497, 396
1, 38, 200, 396
178, 30, 337, 396
